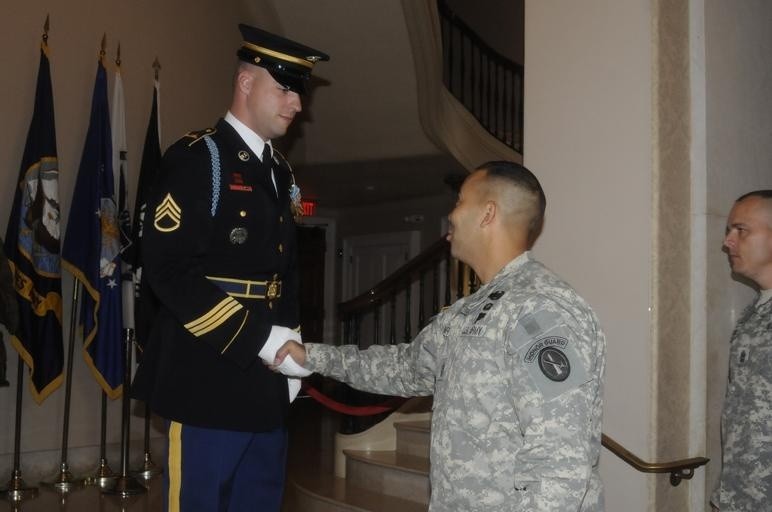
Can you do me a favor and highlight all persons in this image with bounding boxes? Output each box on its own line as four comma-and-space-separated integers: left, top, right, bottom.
263, 161, 607, 512
710, 190, 772, 512
131, 24, 328, 512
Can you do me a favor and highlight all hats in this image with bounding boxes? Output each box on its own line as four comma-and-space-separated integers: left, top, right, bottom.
236, 23, 330, 97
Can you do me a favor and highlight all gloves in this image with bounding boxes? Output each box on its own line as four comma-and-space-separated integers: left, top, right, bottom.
258, 325, 314, 404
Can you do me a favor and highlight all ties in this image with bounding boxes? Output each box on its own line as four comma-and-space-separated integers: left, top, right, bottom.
263, 143, 271, 178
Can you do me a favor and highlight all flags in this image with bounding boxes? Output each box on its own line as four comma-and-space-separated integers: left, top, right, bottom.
0, 41, 162, 406
